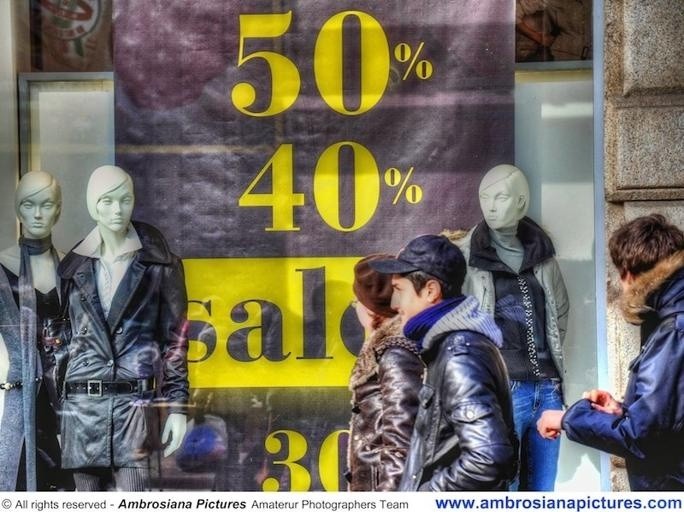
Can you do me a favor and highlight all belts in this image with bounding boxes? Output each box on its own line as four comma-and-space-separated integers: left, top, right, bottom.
64, 379, 156, 395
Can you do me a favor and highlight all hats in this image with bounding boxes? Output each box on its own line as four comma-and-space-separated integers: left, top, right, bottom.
368, 234, 466, 286
353, 254, 400, 318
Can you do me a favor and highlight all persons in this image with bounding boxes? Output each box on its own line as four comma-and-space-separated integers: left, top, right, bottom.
0, 168, 68, 491
368, 233, 522, 491
450, 163, 571, 491
535, 212, 684, 491
347, 252, 426, 491
47, 164, 191, 491
